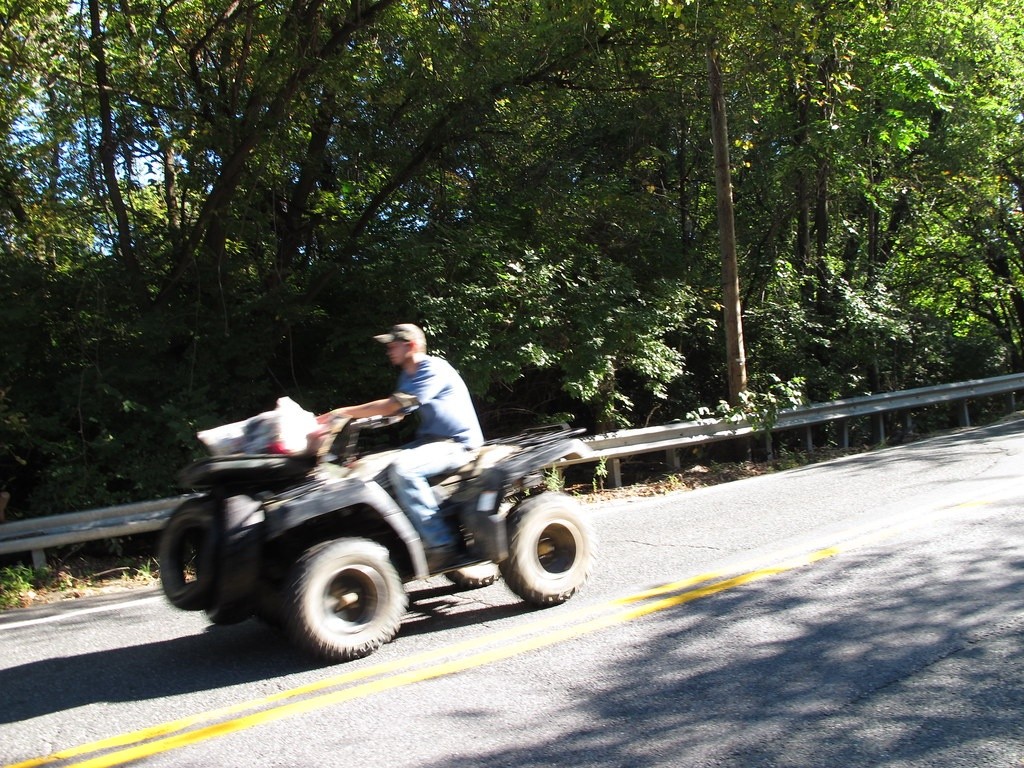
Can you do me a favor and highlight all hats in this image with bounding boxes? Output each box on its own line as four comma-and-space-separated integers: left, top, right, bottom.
373, 323, 427, 347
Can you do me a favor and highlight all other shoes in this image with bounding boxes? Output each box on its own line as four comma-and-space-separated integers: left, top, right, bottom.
427, 546, 470, 574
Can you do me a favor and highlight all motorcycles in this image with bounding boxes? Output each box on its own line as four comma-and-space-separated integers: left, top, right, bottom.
159, 406, 599, 662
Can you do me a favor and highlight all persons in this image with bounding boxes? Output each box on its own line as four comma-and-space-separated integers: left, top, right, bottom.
315, 324, 484, 572
